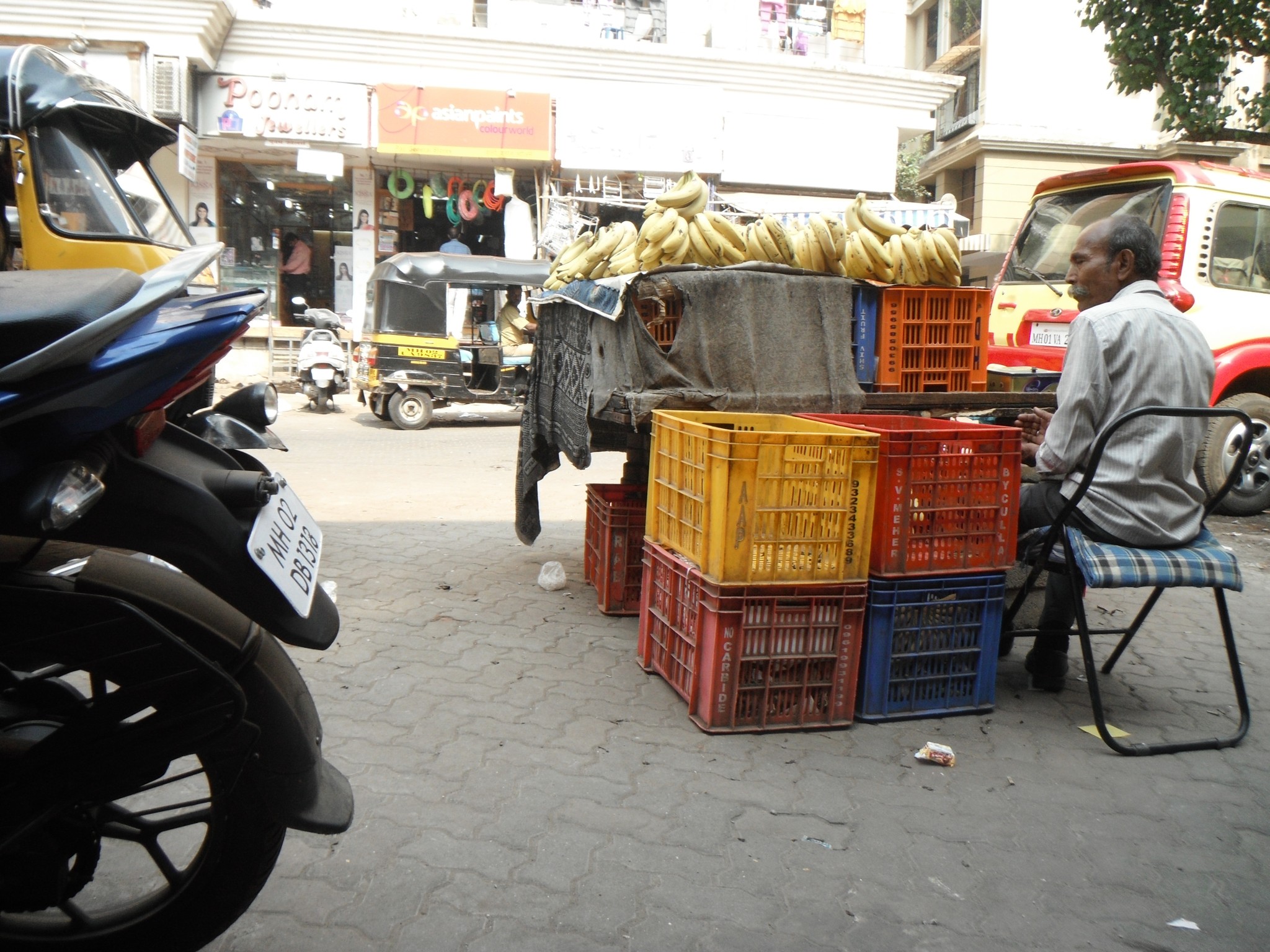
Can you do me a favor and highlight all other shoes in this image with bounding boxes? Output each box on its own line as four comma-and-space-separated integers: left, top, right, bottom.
1025, 649, 1064, 692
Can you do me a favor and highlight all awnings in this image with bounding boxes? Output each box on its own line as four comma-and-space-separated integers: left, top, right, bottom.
712, 189, 958, 237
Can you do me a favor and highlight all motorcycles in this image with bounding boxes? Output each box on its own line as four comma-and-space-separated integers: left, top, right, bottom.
0, 42, 232, 433
354, 254, 564, 430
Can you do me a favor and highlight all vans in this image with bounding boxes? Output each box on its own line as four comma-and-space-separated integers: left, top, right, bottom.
984, 157, 1269, 514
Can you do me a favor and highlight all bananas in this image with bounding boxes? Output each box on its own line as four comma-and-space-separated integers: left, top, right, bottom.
543, 170, 800, 290
791, 192, 966, 287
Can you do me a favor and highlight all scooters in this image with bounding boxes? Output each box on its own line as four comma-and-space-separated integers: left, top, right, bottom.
288, 297, 352, 414
0, 241, 359, 951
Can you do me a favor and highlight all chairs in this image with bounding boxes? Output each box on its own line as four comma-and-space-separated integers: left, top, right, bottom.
996, 403, 1258, 762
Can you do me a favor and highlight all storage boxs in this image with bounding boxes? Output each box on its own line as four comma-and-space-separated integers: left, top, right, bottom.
582, 285, 1026, 738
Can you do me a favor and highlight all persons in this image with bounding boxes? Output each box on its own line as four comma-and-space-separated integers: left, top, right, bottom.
495, 285, 537, 357
439, 228, 474, 338
279, 232, 312, 326
251, 236, 264, 251
999, 216, 1215, 689
189, 202, 217, 227
504, 194, 534, 261
335, 262, 353, 281
353, 209, 375, 230
